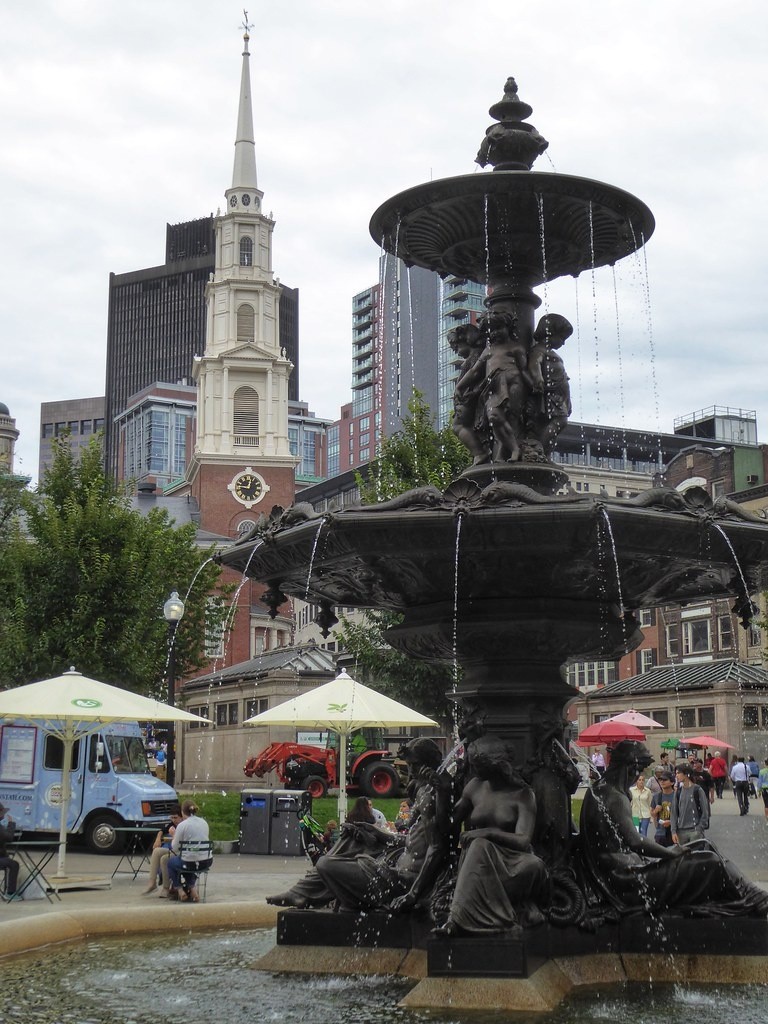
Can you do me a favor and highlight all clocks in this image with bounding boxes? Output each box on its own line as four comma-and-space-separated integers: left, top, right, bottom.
227, 467, 270, 509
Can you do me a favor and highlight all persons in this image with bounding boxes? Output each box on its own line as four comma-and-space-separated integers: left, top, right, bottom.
265, 738, 452, 909
346, 728, 368, 761
0, 803, 23, 901
143, 805, 183, 898
670, 763, 708, 845
447, 309, 574, 467
418, 736, 549, 935
592, 747, 606, 774
149, 740, 167, 765
346, 797, 386, 828
166, 800, 213, 902
399, 799, 413, 813
580, 740, 768, 919
630, 752, 675, 847
687, 751, 768, 816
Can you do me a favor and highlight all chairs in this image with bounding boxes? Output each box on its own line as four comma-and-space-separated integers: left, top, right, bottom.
0, 827, 23, 897
161, 837, 214, 902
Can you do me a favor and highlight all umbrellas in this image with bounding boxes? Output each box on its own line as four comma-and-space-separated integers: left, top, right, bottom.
680, 735, 736, 768
579, 719, 646, 746
243, 668, 440, 831
608, 709, 664, 728
0, 665, 215, 875
575, 740, 606, 747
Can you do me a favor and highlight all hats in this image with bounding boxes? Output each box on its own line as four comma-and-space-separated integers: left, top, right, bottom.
0, 803, 10, 817
690, 758, 703, 764
658, 771, 675, 780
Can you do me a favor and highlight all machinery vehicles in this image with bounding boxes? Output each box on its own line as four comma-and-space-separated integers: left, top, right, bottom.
244, 727, 398, 799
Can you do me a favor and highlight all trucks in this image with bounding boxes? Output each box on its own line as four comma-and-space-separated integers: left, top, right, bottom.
0, 718, 180, 854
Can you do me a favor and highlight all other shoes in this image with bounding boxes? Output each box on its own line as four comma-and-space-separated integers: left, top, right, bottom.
740, 809, 747, 815
159, 889, 170, 898
142, 886, 158, 894
180, 895, 188, 901
4, 891, 22, 901
192, 895, 200, 902
717, 795, 719, 798
720, 795, 722, 799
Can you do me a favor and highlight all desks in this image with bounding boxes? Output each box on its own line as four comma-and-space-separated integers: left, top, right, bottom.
5, 841, 68, 904
111, 827, 162, 880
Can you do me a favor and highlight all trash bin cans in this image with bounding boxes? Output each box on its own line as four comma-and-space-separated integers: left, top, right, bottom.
271, 789, 314, 856
239, 788, 275, 855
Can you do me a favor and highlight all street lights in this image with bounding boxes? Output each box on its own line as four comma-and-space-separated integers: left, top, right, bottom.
163, 591, 185, 787
